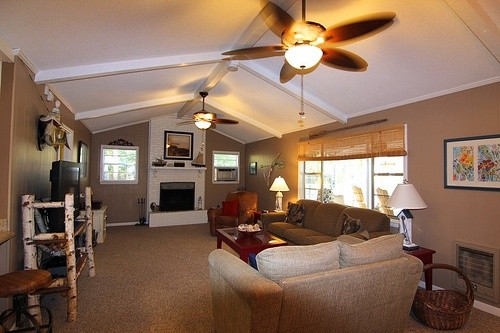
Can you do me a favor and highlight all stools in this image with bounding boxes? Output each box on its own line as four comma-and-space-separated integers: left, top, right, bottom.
0, 269, 52, 333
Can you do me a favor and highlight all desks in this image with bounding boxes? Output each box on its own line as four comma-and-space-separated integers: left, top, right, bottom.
216, 227, 287, 264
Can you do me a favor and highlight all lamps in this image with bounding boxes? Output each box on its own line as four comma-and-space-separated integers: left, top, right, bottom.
269, 176, 290, 212
192, 92, 214, 145
280, 1, 328, 115
384, 184, 428, 250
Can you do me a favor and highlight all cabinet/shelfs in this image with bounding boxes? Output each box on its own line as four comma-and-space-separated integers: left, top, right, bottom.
79, 205, 108, 243
21, 186, 96, 329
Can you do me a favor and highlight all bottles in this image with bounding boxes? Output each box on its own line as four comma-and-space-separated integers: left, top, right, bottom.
198, 196, 202, 210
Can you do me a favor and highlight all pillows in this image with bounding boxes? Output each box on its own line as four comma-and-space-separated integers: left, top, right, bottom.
341, 213, 363, 235
284, 201, 305, 228
222, 200, 240, 217
338, 234, 404, 270
256, 240, 342, 280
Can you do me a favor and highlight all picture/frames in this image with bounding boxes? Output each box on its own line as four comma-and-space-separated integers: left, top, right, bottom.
444, 134, 500, 191
249, 162, 257, 175
164, 130, 193, 160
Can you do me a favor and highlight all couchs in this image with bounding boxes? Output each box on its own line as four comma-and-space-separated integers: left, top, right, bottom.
260, 199, 391, 246
207, 190, 258, 236
207, 234, 425, 333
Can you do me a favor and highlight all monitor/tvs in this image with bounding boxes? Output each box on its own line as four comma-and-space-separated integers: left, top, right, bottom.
50, 160, 82, 232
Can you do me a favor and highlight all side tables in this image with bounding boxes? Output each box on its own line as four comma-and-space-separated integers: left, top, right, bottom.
403, 246, 436, 291
253, 211, 275, 225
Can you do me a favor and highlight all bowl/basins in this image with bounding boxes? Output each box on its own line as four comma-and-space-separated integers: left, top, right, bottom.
236, 227, 262, 235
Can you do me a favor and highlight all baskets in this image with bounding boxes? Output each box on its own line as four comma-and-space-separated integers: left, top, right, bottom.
412, 263, 475, 330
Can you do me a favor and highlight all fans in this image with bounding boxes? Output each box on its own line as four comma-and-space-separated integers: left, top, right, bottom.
221, 0, 396, 82
170, 115, 240, 130
